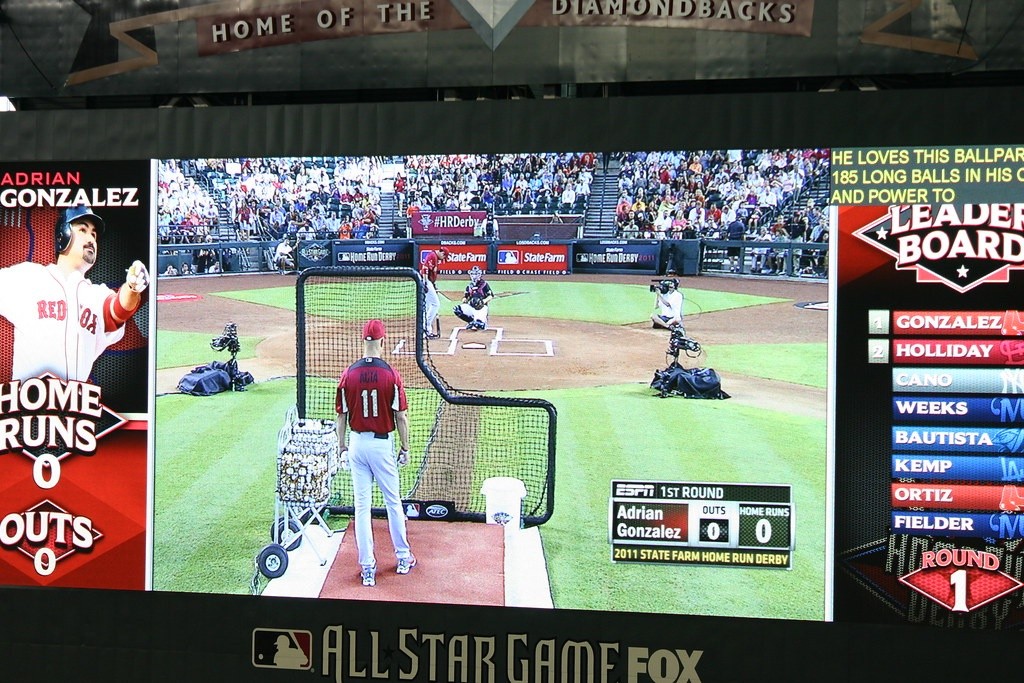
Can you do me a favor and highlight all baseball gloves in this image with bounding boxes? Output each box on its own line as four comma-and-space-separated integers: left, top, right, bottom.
468, 296, 483, 310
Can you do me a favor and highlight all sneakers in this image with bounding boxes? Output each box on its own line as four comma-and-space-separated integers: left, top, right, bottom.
359, 565, 376, 586
396, 552, 416, 574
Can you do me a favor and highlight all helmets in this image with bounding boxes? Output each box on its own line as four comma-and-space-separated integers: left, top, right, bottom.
53, 204, 103, 254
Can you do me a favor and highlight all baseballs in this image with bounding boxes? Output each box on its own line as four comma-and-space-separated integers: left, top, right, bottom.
276, 416, 340, 504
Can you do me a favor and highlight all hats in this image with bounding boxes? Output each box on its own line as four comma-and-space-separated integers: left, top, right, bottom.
441, 246, 449, 263
361, 320, 388, 341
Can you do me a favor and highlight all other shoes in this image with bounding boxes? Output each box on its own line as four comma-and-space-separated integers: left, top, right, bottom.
428, 333, 438, 338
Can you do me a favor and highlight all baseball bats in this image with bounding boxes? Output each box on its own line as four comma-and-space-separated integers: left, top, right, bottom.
435, 313, 440, 338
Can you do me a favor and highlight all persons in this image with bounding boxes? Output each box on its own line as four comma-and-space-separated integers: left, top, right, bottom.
650, 279, 683, 331
452, 266, 494, 330
335, 319, 417, 587
157, 147, 832, 277
418, 245, 452, 340
0, 207, 149, 383
